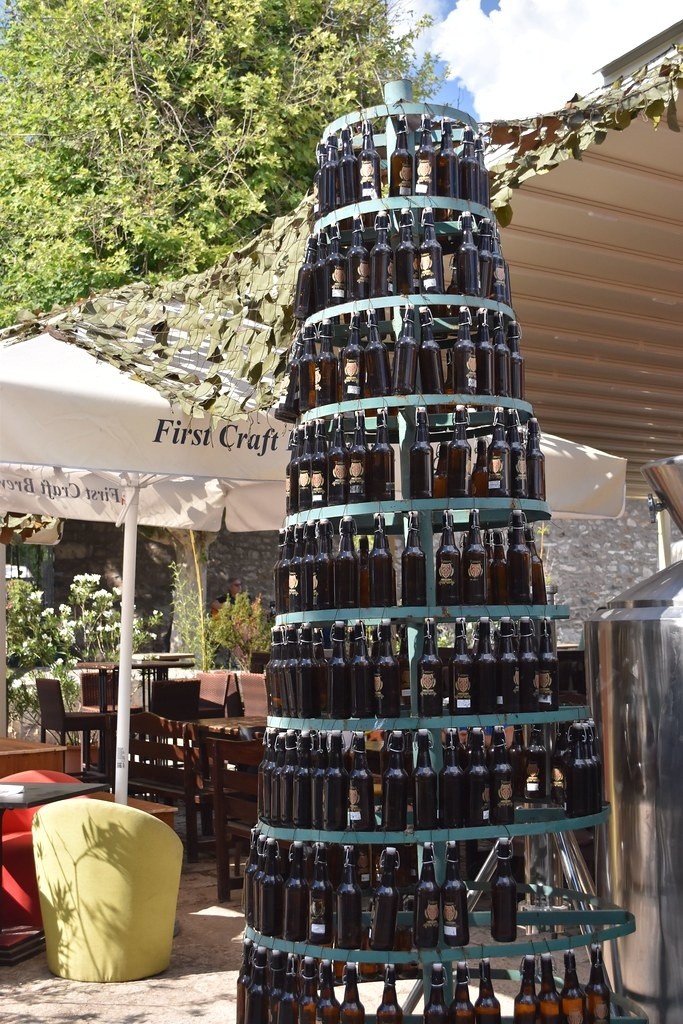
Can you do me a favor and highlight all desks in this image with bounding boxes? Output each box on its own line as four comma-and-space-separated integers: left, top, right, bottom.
189, 715, 267, 836
0, 737, 67, 780
78, 662, 194, 773
136, 653, 195, 765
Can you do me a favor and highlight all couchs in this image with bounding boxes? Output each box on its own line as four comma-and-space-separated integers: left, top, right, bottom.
0, 770, 90, 968
31, 798, 184, 982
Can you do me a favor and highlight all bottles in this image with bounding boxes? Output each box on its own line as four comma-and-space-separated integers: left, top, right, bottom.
335, 845, 363, 950
440, 840, 469, 947
236, 938, 403, 1024
308, 842, 334, 944
314, 120, 382, 222
285, 408, 396, 516
379, 720, 603, 831
257, 726, 375, 832
423, 958, 502, 1024
390, 114, 490, 210
392, 303, 525, 400
401, 509, 548, 607
514, 943, 610, 1024
410, 405, 546, 501
491, 838, 517, 942
446, 211, 512, 317
244, 828, 283, 938
417, 617, 559, 718
294, 207, 445, 321
368, 847, 400, 951
274, 309, 392, 424
413, 842, 441, 949
274, 512, 396, 616
265, 618, 411, 720
283, 841, 309, 942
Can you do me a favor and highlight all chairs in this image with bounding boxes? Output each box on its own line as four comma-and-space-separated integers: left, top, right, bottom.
36, 673, 267, 903
